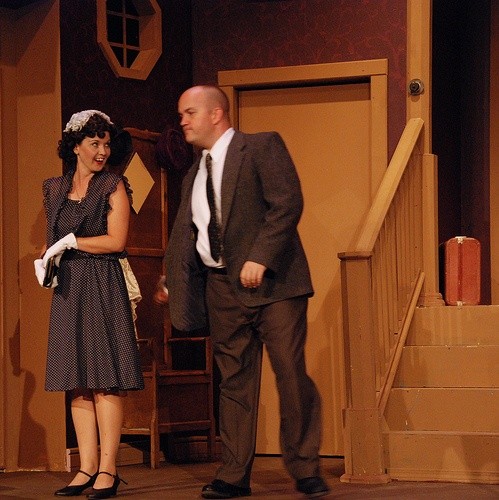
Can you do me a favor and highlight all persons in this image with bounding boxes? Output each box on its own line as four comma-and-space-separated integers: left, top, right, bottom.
34, 109, 144, 500
165, 85, 330, 500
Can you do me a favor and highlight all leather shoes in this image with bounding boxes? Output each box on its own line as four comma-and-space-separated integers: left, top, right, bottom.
87, 470, 120, 500
201, 478, 251, 498
295, 477, 329, 498
55, 470, 99, 496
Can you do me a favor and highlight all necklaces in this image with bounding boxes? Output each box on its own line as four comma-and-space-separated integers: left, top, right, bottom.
72, 177, 95, 203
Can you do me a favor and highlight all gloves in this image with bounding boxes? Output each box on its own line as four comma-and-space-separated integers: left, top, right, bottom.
43, 233, 79, 269
33, 258, 58, 289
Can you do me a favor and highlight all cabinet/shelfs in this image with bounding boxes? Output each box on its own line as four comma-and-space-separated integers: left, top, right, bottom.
121, 336, 216, 469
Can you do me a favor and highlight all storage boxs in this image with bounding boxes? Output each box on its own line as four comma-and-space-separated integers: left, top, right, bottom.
444, 237, 482, 306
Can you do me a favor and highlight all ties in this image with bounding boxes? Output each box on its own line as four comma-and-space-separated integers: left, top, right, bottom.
204, 153, 224, 264
